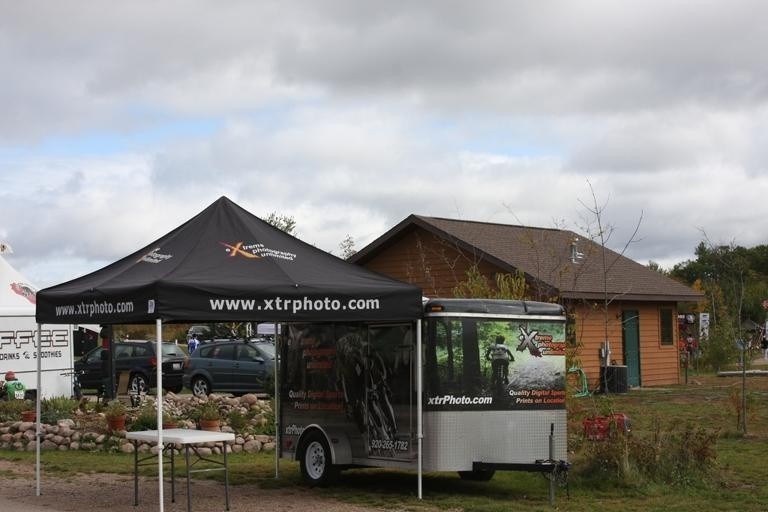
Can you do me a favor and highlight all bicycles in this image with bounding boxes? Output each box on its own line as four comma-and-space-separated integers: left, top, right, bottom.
488, 359, 515, 387
352, 378, 397, 457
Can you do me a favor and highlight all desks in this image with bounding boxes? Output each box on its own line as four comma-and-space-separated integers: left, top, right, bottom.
123, 426, 236, 512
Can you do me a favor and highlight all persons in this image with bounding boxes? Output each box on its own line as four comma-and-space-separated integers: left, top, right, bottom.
338, 335, 399, 454
2, 372, 26, 401
679, 334, 694, 354
486, 335, 515, 390
188, 334, 200, 355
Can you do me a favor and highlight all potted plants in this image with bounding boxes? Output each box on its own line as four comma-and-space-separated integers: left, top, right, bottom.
104, 402, 221, 436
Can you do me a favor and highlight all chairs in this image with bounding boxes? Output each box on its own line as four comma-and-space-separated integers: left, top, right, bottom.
102, 350, 110, 361
239, 349, 253, 362
118, 352, 129, 357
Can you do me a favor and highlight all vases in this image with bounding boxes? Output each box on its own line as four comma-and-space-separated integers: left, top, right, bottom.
21, 409, 36, 422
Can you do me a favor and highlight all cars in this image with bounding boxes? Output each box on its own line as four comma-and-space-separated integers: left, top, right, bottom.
73, 331, 94, 356
74, 339, 188, 399
186, 326, 210, 343
183, 338, 281, 399
